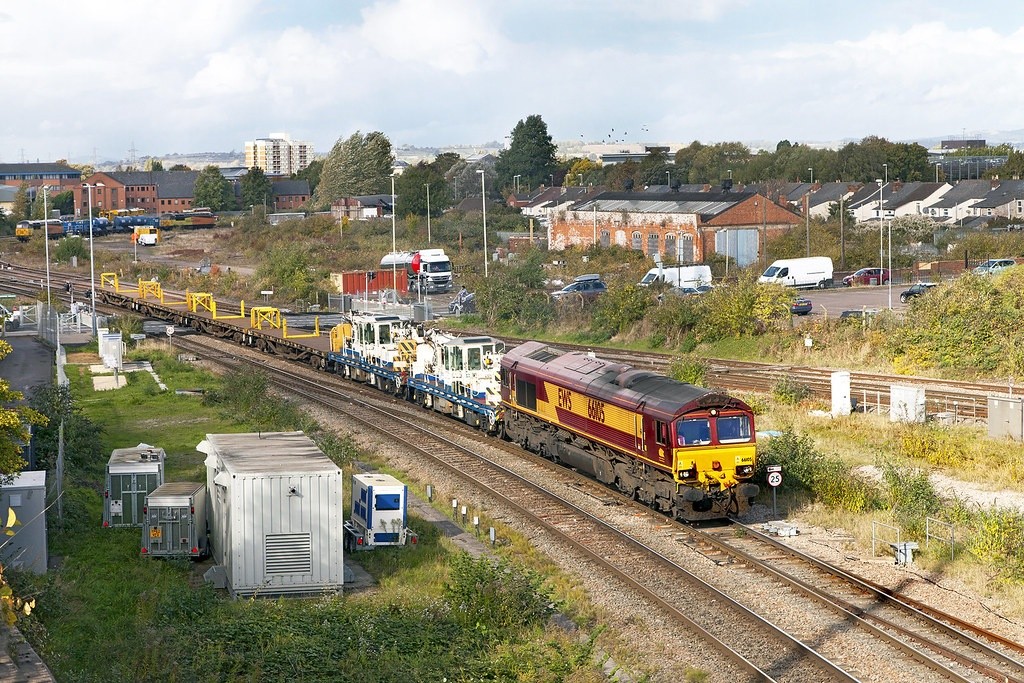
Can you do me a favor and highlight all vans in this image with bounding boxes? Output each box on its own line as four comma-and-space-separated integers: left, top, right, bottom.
758, 255, 836, 291
136, 227, 158, 246
636, 265, 714, 291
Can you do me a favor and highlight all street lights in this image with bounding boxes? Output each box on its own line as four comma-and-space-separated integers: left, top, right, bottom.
514, 174, 522, 193
423, 182, 431, 247
886, 217, 890, 310
388, 173, 396, 305
83, 182, 96, 334
475, 168, 488, 277
43, 186, 49, 318
722, 228, 728, 283
875, 177, 879, 285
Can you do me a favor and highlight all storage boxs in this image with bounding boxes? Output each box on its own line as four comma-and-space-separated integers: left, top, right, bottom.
196, 430, 344, 597
330, 268, 408, 295
139, 482, 206, 559
103, 448, 165, 529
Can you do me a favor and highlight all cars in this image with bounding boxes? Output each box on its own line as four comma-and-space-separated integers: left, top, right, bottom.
842, 266, 895, 285
968, 257, 1020, 279
656, 285, 714, 304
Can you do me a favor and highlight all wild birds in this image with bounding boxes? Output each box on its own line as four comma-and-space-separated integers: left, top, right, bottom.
641, 125, 649, 132
603, 129, 627, 142
581, 135, 583, 137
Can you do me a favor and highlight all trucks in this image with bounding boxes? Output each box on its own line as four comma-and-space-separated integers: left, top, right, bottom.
380, 248, 455, 293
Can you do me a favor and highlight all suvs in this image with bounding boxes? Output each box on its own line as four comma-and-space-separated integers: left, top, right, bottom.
899, 283, 938, 303
551, 279, 608, 299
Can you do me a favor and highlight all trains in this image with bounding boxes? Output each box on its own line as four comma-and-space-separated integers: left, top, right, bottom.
65, 277, 760, 522
15, 207, 215, 242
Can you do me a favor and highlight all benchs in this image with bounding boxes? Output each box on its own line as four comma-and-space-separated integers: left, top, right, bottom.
891, 542, 919, 563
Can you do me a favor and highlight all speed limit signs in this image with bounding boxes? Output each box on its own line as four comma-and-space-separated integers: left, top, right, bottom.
766, 472, 783, 486
167, 328, 174, 335
